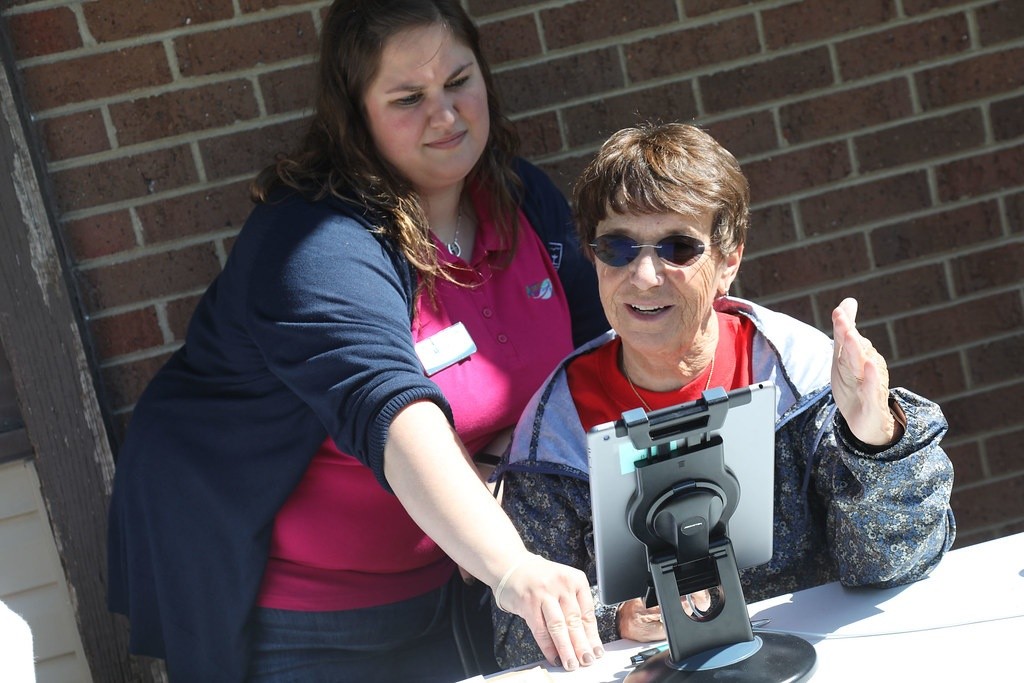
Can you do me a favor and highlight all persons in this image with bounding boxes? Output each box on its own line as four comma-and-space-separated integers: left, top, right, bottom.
491, 123, 955, 672
107, 0, 613, 682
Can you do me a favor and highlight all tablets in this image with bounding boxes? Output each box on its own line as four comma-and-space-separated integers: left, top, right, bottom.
587, 379, 776, 607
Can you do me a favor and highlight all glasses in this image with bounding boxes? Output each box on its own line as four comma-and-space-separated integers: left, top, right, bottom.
590, 232, 716, 267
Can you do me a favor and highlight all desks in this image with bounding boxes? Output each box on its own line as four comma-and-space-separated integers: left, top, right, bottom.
453, 531, 1024, 683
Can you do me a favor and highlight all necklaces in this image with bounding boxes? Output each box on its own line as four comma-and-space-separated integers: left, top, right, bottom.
623, 357, 716, 411
447, 214, 463, 256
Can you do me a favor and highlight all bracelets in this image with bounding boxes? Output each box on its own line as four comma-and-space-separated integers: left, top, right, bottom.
495, 555, 537, 612
